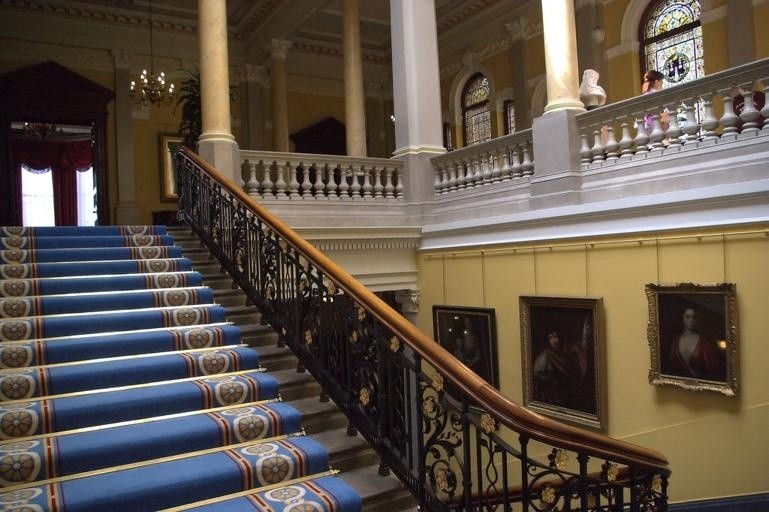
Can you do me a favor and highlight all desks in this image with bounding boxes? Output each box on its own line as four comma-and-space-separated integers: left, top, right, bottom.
151, 211, 178, 226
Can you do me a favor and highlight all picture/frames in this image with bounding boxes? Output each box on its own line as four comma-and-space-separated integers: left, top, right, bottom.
157, 132, 189, 203
644, 280, 740, 400
431, 303, 501, 431
519, 295, 608, 433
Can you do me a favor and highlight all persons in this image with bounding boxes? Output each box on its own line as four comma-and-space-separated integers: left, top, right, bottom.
531, 328, 575, 407
642, 70, 671, 133
668, 302, 713, 377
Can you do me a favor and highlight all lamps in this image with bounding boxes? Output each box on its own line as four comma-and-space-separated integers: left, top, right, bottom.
128, 0, 176, 108
